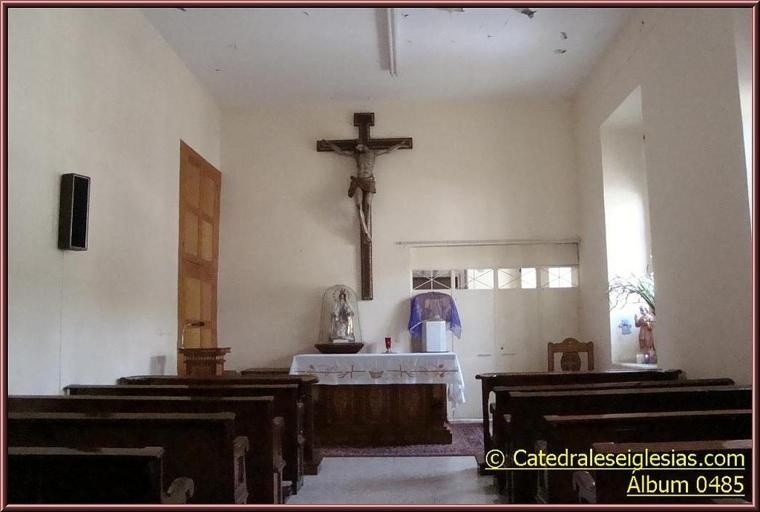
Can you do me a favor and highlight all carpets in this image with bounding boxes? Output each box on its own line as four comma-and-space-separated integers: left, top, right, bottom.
316, 420, 487, 458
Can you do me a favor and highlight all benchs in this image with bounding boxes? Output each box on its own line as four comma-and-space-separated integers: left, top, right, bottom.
8, 369, 326, 506
476, 365, 753, 505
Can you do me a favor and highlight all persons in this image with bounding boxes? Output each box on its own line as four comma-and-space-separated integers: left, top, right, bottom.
634, 303, 654, 352
320, 139, 409, 245
328, 290, 355, 336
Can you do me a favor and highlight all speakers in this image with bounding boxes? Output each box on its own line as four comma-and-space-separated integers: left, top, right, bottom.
58, 173, 91, 251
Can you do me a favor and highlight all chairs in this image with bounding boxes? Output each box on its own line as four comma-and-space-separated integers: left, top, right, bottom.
547, 337, 595, 371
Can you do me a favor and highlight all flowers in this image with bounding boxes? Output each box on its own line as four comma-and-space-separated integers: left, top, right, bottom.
605, 268, 655, 318
